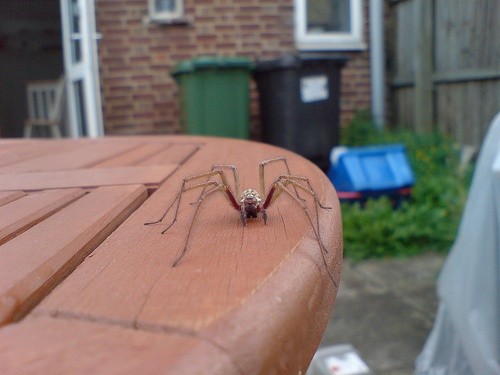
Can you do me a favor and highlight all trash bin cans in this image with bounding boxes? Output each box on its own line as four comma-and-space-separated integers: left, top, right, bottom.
250, 53, 348, 164
328, 142, 416, 212
170, 56, 256, 140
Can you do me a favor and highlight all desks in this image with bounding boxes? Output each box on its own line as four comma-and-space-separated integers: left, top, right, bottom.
0, 134, 345, 375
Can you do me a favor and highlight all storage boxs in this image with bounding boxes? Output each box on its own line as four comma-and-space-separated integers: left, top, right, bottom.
327, 141, 415, 209
